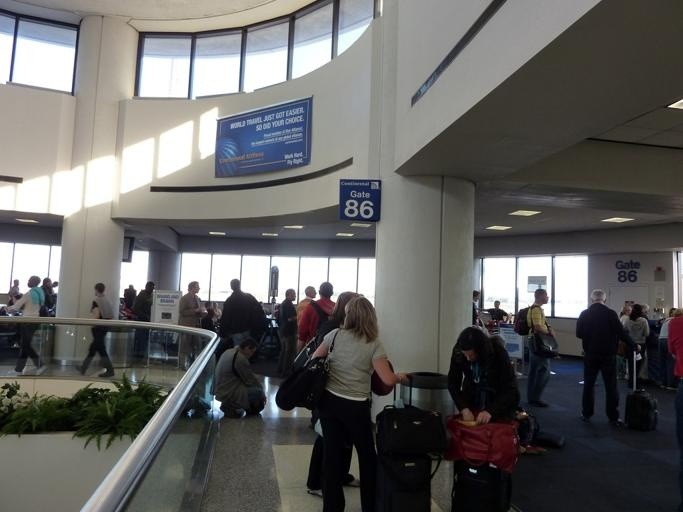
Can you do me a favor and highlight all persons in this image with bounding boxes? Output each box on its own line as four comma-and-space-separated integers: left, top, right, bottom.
448, 327, 519, 424
620, 301, 682, 390
308, 291, 408, 512
221, 279, 251, 346
8, 278, 58, 347
179, 282, 208, 371
210, 337, 261, 417
278, 282, 335, 377
575, 290, 637, 423
7, 277, 47, 375
202, 302, 222, 332
527, 289, 553, 407
75, 283, 115, 377
125, 283, 154, 355
473, 291, 509, 325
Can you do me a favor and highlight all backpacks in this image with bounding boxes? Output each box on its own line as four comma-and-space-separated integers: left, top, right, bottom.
513, 307, 529, 336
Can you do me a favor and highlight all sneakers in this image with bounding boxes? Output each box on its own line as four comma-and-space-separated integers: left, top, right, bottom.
581, 409, 594, 421
220, 402, 241, 419
655, 385, 666, 391
74, 364, 87, 375
608, 418, 626, 425
8, 367, 23, 376
98, 368, 114, 378
526, 400, 550, 408
666, 383, 680, 392
35, 364, 48, 376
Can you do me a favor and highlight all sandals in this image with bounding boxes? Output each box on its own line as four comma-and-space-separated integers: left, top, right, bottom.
304, 485, 323, 498
341, 474, 360, 487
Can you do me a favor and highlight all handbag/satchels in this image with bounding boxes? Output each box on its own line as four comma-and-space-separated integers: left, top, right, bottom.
443, 412, 521, 474
526, 331, 559, 358
375, 405, 416, 470
274, 365, 328, 412
245, 395, 265, 418
38, 304, 50, 319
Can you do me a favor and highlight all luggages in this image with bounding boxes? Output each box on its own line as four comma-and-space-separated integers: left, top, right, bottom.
450, 457, 513, 511
623, 346, 660, 432
374, 375, 432, 511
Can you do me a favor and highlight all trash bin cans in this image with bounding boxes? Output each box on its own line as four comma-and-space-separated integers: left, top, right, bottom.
400, 372, 455, 416
